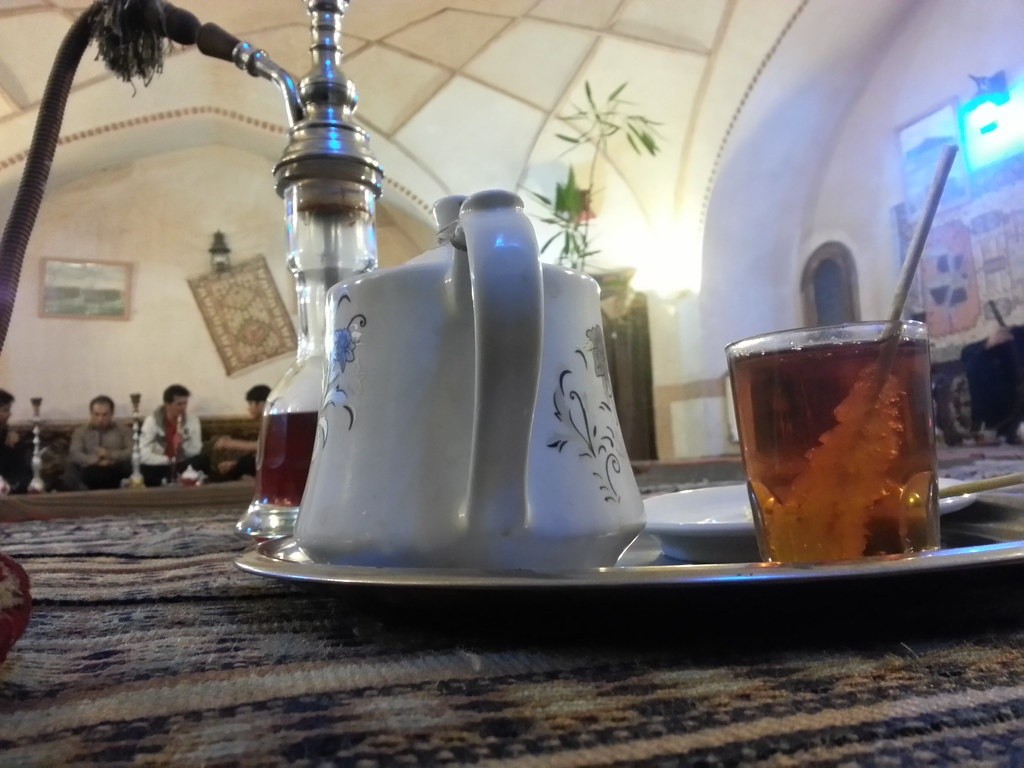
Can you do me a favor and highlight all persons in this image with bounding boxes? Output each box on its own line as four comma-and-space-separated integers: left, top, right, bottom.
0, 387, 20, 468
213, 384, 271, 479
63, 395, 134, 490
137, 385, 211, 487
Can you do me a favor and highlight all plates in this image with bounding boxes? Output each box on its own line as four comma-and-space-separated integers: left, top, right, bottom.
643, 477, 980, 561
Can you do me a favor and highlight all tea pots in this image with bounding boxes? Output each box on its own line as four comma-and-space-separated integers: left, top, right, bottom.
293, 190, 645, 569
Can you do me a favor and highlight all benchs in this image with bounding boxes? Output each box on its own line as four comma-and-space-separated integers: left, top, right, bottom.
7, 417, 258, 483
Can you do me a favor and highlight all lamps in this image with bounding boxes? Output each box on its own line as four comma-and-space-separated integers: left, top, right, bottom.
208, 231, 232, 277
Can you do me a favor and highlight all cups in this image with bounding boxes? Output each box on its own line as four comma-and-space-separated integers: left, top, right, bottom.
726, 320, 942, 565
